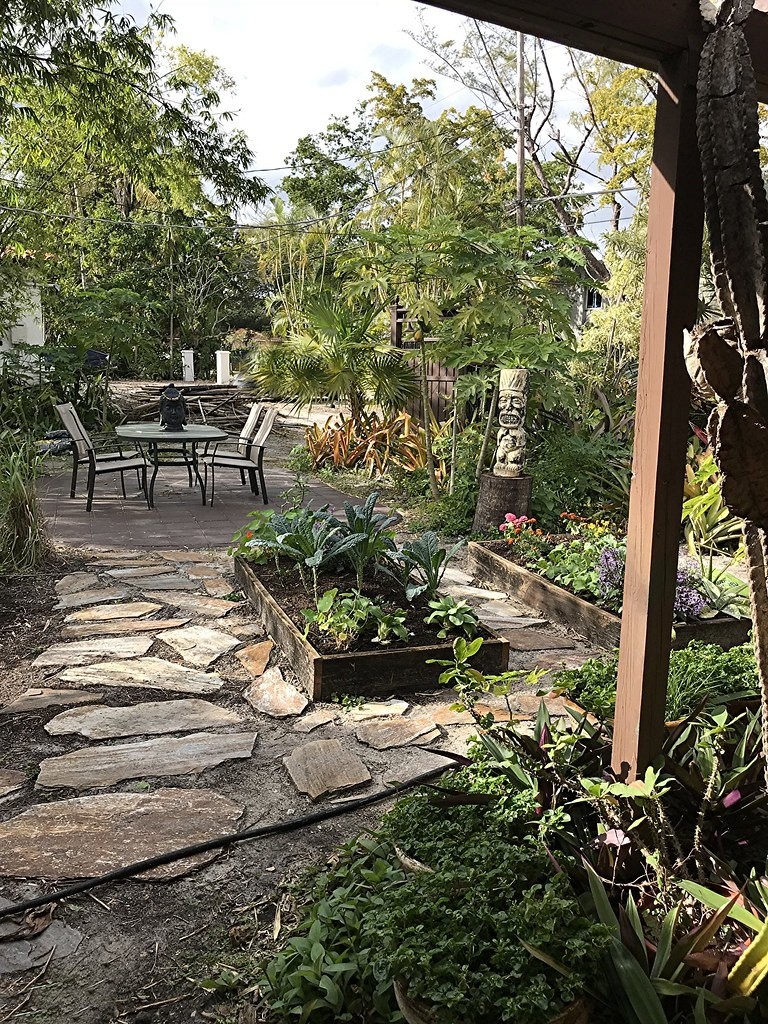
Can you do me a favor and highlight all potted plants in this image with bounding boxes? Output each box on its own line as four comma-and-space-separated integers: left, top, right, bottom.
467, 536, 753, 655
227, 470, 509, 702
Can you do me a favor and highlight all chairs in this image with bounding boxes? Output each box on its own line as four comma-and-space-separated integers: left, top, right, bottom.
53, 402, 141, 500
203, 409, 279, 506
69, 406, 153, 512
194, 403, 265, 498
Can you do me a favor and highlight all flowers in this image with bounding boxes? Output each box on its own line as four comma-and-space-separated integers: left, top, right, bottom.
499, 508, 705, 618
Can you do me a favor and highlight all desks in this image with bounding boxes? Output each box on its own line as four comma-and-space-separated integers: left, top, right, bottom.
115, 424, 229, 507
